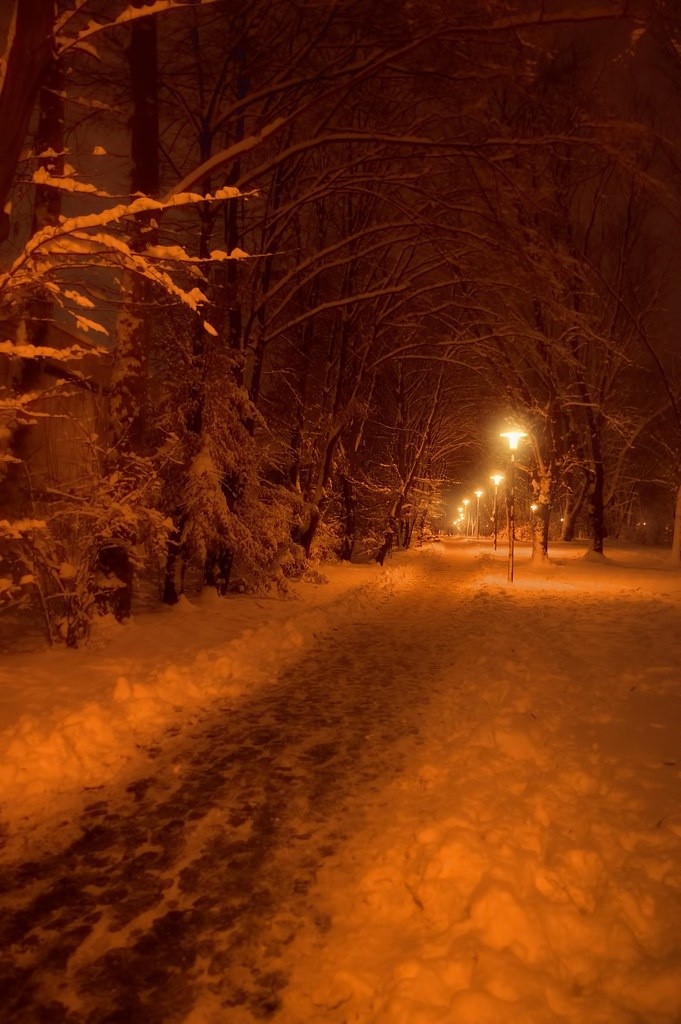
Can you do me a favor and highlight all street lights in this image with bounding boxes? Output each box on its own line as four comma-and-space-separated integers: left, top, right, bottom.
501, 431, 528, 586
491, 475, 505, 551
474, 491, 484, 540
463, 500, 470, 538
